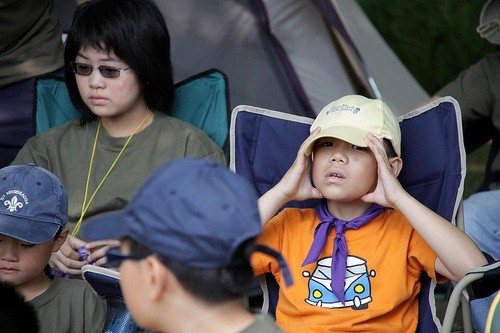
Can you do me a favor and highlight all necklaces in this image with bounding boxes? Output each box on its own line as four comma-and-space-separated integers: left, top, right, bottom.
71, 111, 152, 238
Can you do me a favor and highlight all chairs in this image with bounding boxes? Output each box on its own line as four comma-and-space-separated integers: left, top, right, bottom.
81, 96, 500, 333
29, 68, 232, 172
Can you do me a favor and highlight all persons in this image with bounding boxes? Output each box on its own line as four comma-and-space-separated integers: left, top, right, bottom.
6, 1, 227, 235
82, 157, 280, 332
0, 280, 42, 332
0, 162, 107, 333
432, 0, 500, 300
248, 96, 488, 333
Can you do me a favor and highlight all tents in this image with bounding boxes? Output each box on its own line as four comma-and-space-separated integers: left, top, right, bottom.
1, 0, 432, 172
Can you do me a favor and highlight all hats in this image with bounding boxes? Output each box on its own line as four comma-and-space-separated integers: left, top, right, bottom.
81, 157, 293, 287
0, 163, 69, 245
476, 0, 500, 44
304, 95, 401, 158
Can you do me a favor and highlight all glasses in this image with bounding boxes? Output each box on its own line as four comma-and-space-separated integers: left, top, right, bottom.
69, 60, 131, 78
